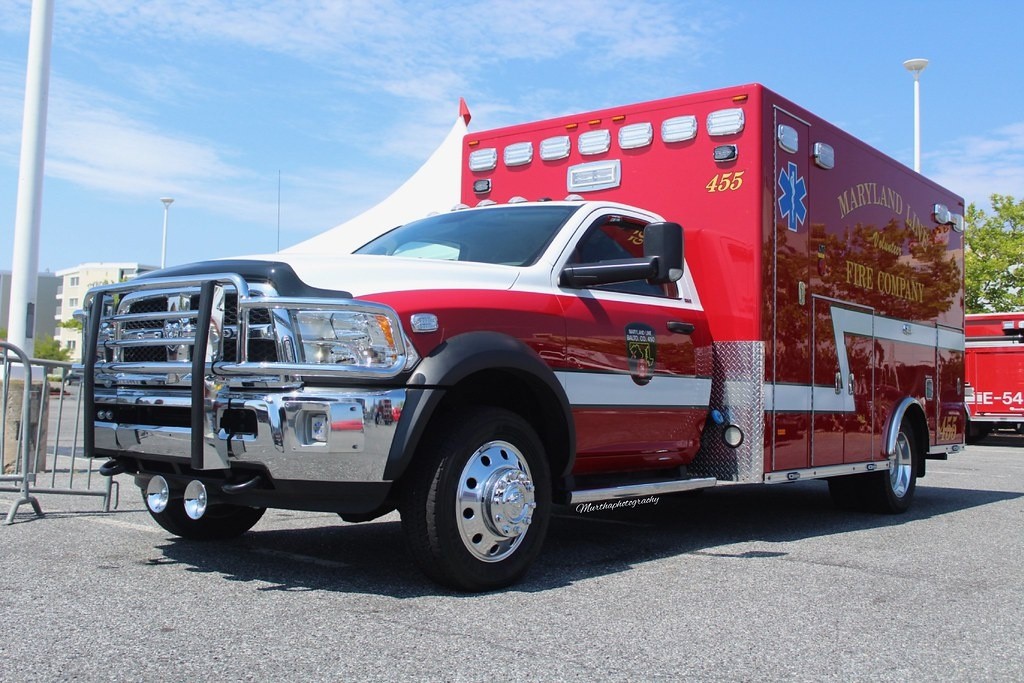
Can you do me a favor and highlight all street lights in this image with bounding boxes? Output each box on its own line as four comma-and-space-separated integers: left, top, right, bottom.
159, 197, 174, 271
903, 58, 929, 173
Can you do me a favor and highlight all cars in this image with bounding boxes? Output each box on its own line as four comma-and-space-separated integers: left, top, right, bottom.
66, 362, 84, 385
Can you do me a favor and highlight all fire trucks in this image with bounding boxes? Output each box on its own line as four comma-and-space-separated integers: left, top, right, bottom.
963, 314, 1024, 444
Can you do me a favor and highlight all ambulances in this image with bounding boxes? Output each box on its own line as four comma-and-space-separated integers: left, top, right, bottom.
80, 82, 967, 593
